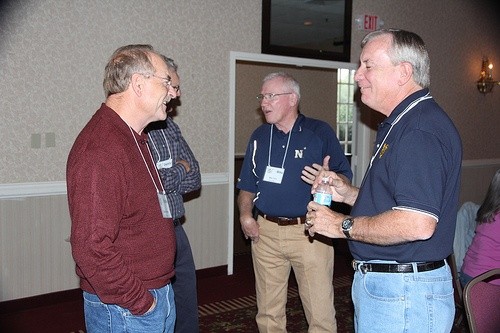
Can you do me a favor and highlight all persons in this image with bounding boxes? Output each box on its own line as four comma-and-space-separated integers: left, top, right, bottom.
294, 28, 463, 333
134, 53, 203, 333
64, 44, 177, 333
234, 71, 354, 333
459, 167, 500, 333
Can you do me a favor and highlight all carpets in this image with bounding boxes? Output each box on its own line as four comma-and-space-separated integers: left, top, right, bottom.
68, 255, 472, 333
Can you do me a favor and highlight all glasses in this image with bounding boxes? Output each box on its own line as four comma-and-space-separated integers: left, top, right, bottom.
144, 73, 172, 90
256, 92, 292, 102
174, 85, 180, 91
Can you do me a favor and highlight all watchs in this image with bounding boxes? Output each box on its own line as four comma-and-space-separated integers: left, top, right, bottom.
341, 216, 356, 241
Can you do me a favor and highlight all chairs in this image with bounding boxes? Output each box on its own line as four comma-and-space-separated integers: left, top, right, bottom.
450, 200, 482, 327
463, 267, 500, 333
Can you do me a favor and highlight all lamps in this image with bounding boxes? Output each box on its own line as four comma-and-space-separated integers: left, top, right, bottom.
475, 53, 500, 97
353, 14, 384, 33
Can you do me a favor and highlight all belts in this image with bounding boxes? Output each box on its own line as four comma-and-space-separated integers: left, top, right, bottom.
257, 210, 305, 227
351, 259, 446, 274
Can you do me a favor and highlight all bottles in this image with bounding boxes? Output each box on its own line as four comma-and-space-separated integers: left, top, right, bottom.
312, 177, 332, 212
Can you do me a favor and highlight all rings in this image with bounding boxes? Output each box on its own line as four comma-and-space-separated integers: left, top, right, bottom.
304, 217, 315, 230
